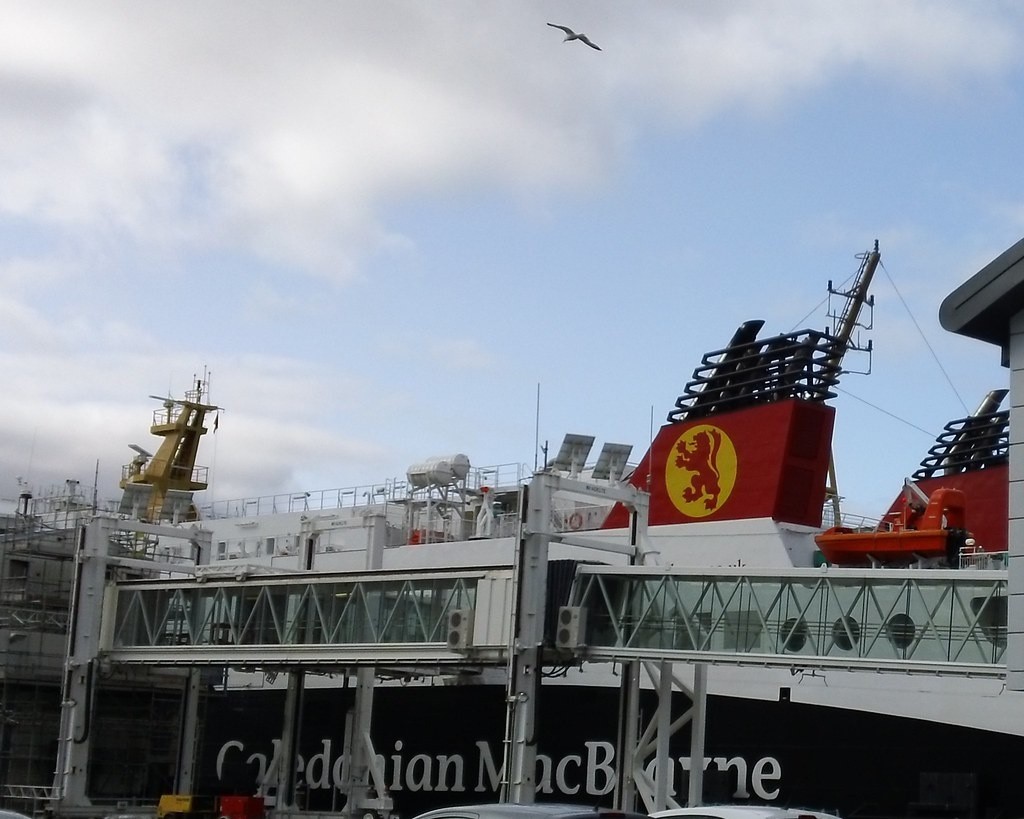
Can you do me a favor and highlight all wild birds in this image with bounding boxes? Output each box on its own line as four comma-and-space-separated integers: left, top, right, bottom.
546, 22, 603, 51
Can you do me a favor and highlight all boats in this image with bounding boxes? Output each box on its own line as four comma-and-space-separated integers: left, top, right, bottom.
814, 524, 953, 566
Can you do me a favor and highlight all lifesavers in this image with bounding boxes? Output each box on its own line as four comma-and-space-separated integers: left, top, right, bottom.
569, 513, 583, 530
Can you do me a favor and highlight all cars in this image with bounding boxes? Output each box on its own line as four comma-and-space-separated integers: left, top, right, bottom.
406, 802, 847, 819
